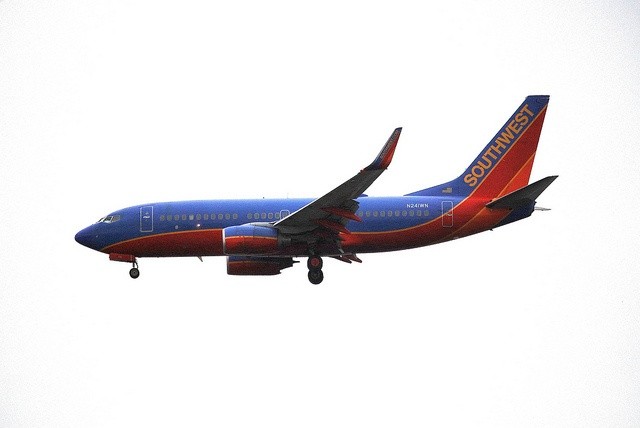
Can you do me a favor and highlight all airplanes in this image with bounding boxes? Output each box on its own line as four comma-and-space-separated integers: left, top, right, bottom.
75, 95, 559, 284
